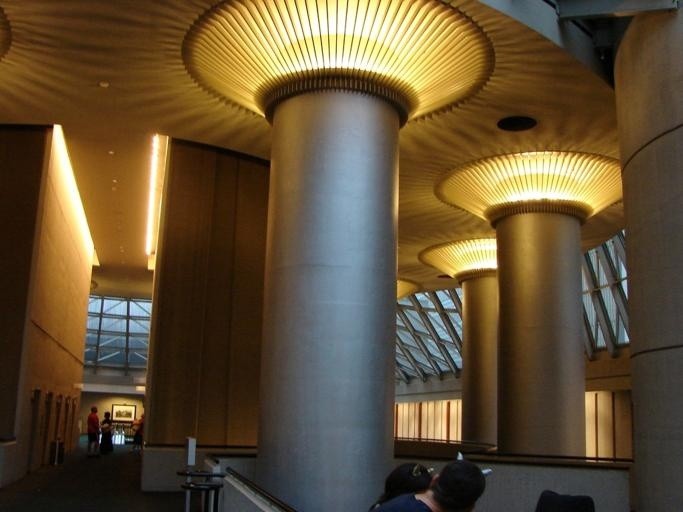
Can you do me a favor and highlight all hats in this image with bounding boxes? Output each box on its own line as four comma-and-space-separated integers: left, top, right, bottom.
375, 461, 434, 502
434, 451, 494, 510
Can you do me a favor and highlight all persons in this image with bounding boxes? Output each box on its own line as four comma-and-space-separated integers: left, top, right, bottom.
367, 461, 433, 511
375, 458, 492, 511
127, 413, 143, 455
86, 405, 100, 459
99, 411, 115, 458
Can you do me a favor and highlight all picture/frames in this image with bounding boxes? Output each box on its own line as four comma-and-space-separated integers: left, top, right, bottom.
111, 404, 136, 423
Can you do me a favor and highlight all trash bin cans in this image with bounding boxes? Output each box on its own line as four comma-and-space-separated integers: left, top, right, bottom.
180, 482, 224, 512
51, 441, 65, 465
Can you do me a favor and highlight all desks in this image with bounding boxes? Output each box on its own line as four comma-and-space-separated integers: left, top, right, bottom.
176, 464, 227, 512
